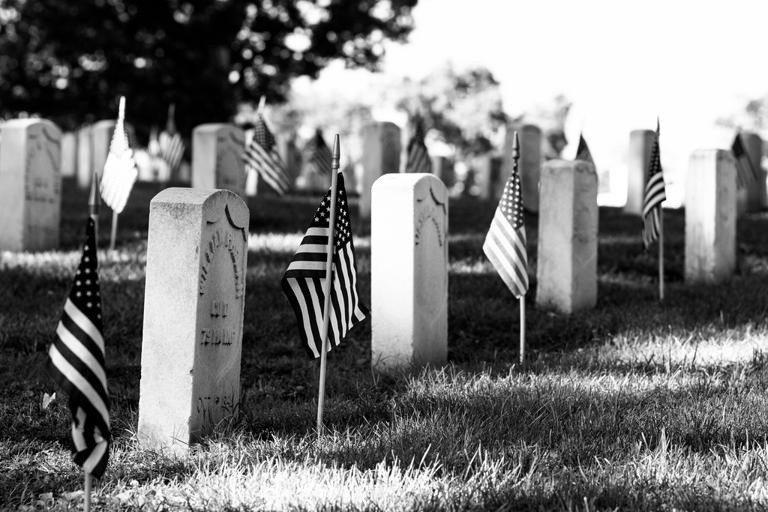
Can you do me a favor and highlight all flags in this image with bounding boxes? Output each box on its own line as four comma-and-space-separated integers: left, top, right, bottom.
148, 129, 184, 181
482, 154, 534, 304
276, 160, 370, 366
727, 130, 759, 193
639, 126, 666, 254
575, 136, 592, 163
406, 125, 432, 174
96, 94, 140, 219
241, 118, 334, 197
45, 210, 114, 482
